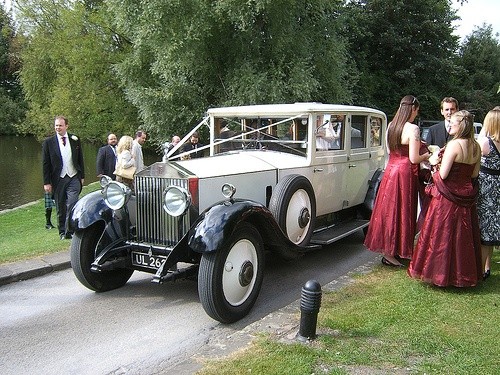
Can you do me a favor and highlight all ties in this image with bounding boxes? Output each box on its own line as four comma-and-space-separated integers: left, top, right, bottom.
193, 145, 196, 155
333, 123, 339, 132
62, 137, 66, 147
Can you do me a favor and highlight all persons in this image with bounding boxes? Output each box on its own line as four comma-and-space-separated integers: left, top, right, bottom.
426, 97, 459, 148
279, 114, 361, 151
476, 106, 500, 279
114, 135, 136, 188
45, 191, 56, 228
132, 131, 147, 173
407, 110, 483, 288
41, 116, 85, 240
363, 96, 432, 269
96, 134, 118, 182
182, 134, 205, 159
167, 136, 182, 161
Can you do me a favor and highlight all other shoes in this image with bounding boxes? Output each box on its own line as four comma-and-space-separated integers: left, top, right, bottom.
66, 234, 73, 239
61, 236, 65, 240
482, 270, 490, 280
382, 257, 405, 268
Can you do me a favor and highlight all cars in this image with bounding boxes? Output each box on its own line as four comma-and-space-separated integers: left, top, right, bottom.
67, 101, 388, 322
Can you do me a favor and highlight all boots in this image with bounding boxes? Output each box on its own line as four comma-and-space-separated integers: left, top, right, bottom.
46, 208, 55, 229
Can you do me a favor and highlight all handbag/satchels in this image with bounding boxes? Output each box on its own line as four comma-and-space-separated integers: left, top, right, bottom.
113, 150, 136, 179
